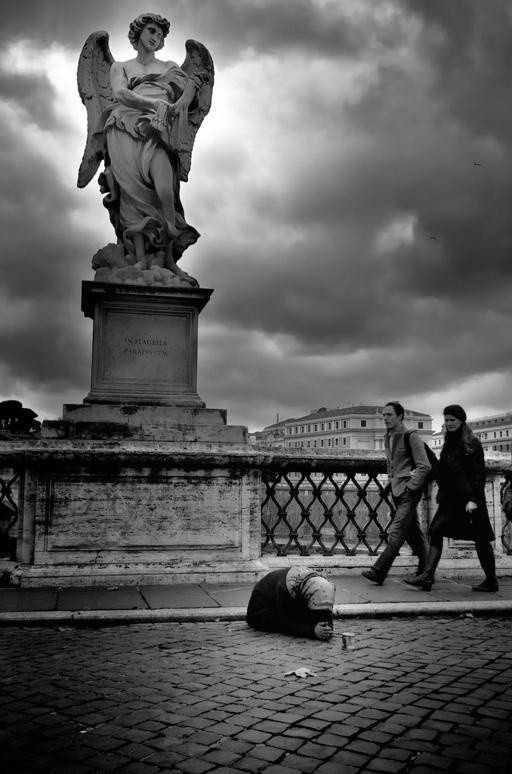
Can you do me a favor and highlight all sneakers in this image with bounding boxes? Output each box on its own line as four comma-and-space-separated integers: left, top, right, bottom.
361, 570, 385, 583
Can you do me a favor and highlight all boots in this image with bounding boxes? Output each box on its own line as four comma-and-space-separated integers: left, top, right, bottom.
472, 543, 498, 592
403, 546, 442, 591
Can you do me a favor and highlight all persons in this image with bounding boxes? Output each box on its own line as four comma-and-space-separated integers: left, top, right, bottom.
405, 404, 498, 591
359, 400, 431, 585
71, 11, 220, 266
246, 566, 335, 641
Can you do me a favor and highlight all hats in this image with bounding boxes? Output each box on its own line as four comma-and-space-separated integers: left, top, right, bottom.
443, 405, 466, 422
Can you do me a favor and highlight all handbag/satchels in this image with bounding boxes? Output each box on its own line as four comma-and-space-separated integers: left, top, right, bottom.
404, 429, 438, 484
